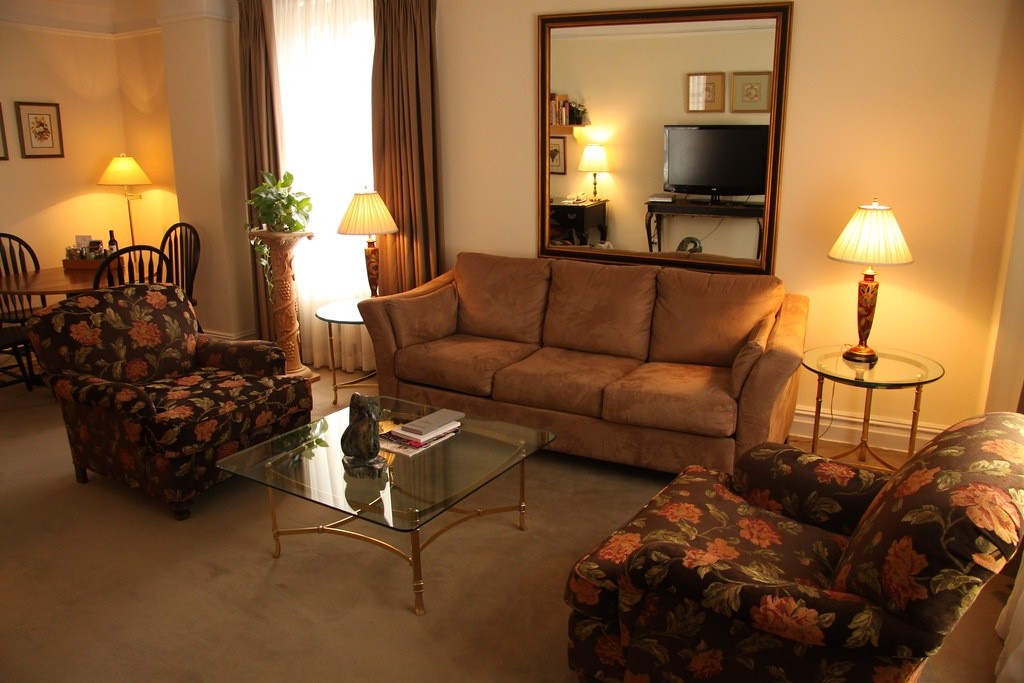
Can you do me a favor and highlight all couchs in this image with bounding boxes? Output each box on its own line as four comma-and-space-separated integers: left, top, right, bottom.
358, 253, 810, 473
24, 282, 313, 519
565, 410, 1023, 683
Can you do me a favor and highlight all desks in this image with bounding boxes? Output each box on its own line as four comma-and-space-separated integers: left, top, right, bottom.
800, 345, 945, 475
315, 303, 378, 405
0, 268, 157, 390
643, 199, 765, 260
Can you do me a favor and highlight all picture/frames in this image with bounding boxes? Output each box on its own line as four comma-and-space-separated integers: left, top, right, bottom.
687, 72, 725, 112
550, 136, 567, 175
731, 71, 772, 113
15, 102, 64, 158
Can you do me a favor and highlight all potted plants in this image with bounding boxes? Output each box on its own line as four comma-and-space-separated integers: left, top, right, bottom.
240, 170, 312, 303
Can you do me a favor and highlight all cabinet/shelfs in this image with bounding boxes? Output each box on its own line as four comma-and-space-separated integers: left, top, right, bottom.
549, 200, 607, 246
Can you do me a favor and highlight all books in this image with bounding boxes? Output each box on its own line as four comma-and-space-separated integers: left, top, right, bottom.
385, 404, 465, 456
548, 99, 571, 126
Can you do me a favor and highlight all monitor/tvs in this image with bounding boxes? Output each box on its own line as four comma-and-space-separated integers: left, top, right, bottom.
662, 125, 770, 206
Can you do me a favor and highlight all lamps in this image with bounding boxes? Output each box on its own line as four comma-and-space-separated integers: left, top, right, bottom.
336, 186, 399, 296
578, 145, 609, 201
828, 198, 914, 363
96, 153, 153, 262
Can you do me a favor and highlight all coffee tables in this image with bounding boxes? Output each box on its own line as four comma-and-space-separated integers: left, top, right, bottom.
216, 396, 557, 616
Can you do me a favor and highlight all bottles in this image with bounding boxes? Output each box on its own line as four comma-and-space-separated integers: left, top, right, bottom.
109, 229, 118, 254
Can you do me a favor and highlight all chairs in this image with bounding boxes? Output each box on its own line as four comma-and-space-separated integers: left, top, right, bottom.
157, 223, 203, 333
94, 245, 171, 291
0, 233, 47, 391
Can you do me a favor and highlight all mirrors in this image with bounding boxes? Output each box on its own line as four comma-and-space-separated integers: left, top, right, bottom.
537, 2, 794, 274
